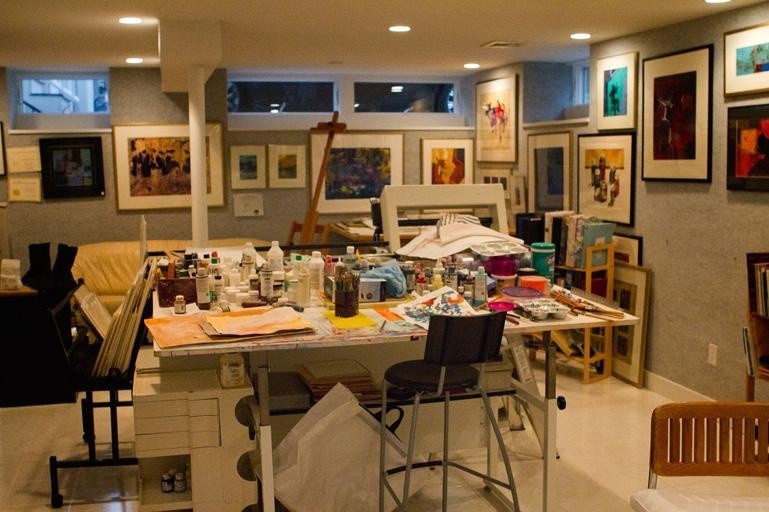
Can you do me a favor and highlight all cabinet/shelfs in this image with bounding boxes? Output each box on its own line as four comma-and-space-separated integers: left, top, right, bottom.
132, 369, 261, 512
528, 244, 617, 383
744, 249, 769, 404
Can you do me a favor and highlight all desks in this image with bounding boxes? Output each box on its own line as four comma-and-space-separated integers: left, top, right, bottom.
148, 266, 640, 512
629, 487, 769, 511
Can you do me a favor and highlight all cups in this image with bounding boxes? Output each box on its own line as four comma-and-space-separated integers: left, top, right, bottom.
530, 242, 556, 286
334, 287, 359, 317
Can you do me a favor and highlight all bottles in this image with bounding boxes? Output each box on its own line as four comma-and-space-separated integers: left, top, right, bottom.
187, 236, 325, 310
399, 255, 487, 308
173, 295, 186, 315
161, 467, 186, 492
340, 244, 369, 278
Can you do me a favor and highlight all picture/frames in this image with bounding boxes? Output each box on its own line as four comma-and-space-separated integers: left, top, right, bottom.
724, 21, 769, 102
526, 130, 573, 212
37, 136, 105, 202
269, 144, 307, 189
728, 103, 768, 193
474, 74, 519, 164
614, 233, 645, 268
597, 51, 639, 131
233, 192, 264, 217
577, 133, 635, 227
641, 42, 714, 183
612, 258, 655, 389
110, 121, 228, 214
309, 132, 527, 243
231, 145, 266, 190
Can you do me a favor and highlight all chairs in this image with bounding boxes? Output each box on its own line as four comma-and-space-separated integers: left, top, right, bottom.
647, 402, 769, 488
383, 311, 523, 512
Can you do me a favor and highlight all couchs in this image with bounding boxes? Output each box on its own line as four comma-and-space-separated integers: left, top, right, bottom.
69, 240, 282, 344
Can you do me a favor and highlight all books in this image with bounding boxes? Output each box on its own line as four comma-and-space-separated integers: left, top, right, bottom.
543, 210, 616, 270
